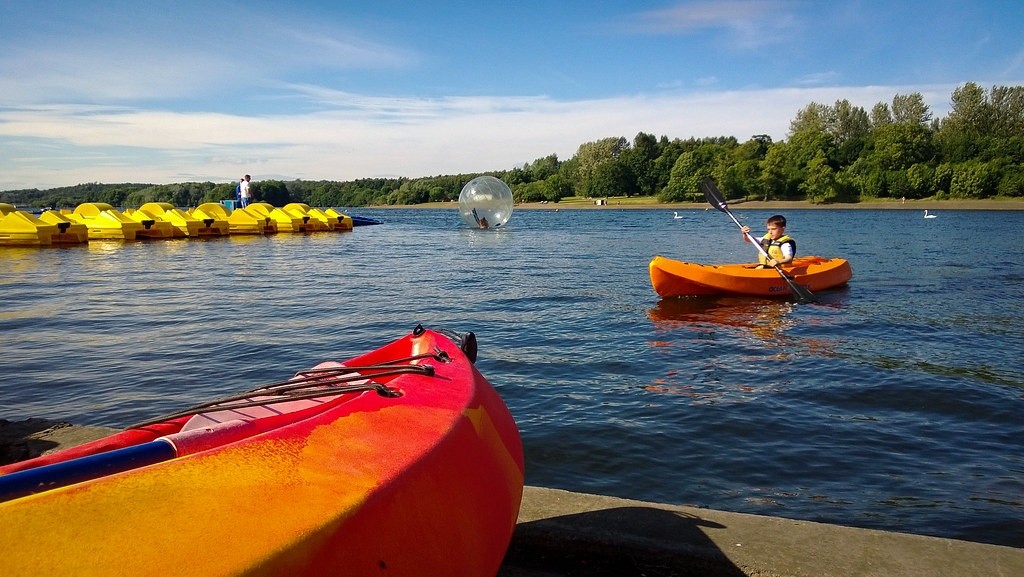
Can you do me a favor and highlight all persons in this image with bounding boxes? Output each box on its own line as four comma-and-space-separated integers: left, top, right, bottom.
742, 215, 797, 270
480, 217, 488, 227
236, 175, 252, 208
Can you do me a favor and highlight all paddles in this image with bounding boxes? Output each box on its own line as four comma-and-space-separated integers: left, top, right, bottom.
0, 360, 377, 502
701, 176, 821, 306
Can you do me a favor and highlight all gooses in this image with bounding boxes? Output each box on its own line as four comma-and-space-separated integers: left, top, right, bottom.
924, 210, 938, 218
674, 212, 682, 218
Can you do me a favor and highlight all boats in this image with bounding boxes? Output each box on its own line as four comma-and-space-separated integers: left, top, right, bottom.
53, 199, 173, 241
650, 256, 853, 297
232, 199, 297, 233
0, 326, 530, 577
0, 200, 89, 245
278, 200, 354, 234
129, 198, 230, 237
